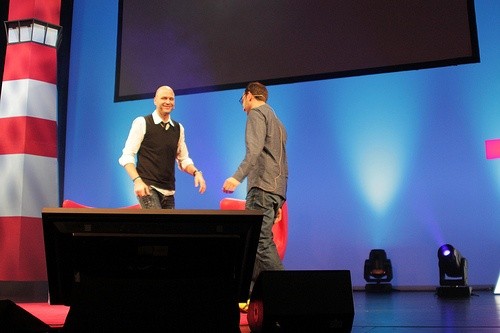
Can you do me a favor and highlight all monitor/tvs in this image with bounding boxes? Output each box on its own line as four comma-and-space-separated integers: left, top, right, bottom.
41, 207, 264, 305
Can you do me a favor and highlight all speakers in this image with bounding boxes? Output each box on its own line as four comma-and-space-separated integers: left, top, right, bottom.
247, 270, 354, 333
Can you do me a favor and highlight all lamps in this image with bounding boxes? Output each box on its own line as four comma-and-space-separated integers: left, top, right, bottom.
436, 244, 472, 298
364, 249, 394, 293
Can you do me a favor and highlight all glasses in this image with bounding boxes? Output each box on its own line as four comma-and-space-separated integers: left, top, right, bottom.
238, 93, 252, 104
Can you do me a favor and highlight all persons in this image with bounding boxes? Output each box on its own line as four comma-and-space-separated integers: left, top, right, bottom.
119, 86, 206, 209
222, 83, 287, 313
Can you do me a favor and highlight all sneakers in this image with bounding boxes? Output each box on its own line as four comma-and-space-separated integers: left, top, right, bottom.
238, 298, 250, 313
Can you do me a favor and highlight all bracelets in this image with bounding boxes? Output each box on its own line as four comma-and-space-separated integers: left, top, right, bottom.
133, 176, 141, 181
194, 169, 202, 176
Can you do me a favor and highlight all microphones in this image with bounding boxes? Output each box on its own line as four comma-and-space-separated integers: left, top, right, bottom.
243, 108, 245, 111
